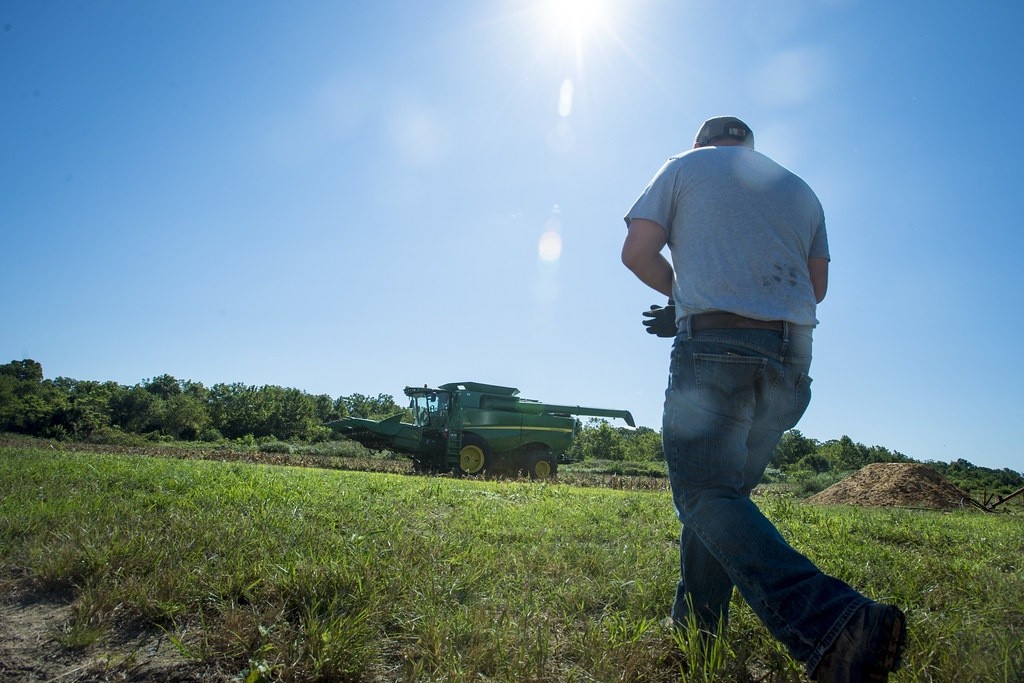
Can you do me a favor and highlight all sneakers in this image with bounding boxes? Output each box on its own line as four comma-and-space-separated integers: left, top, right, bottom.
660, 616, 674, 630
815, 602, 906, 683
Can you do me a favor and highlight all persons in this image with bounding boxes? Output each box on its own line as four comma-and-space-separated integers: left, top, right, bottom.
621, 117, 908, 683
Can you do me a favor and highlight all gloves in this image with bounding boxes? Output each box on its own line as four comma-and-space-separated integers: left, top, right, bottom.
642, 305, 677, 337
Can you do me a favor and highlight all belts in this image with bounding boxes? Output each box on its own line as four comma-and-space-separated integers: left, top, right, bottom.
676, 311, 813, 341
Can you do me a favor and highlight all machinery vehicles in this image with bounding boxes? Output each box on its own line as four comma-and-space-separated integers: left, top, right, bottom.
321, 381, 637, 484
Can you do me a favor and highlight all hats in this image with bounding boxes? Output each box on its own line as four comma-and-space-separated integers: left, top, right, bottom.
695, 117, 755, 147
